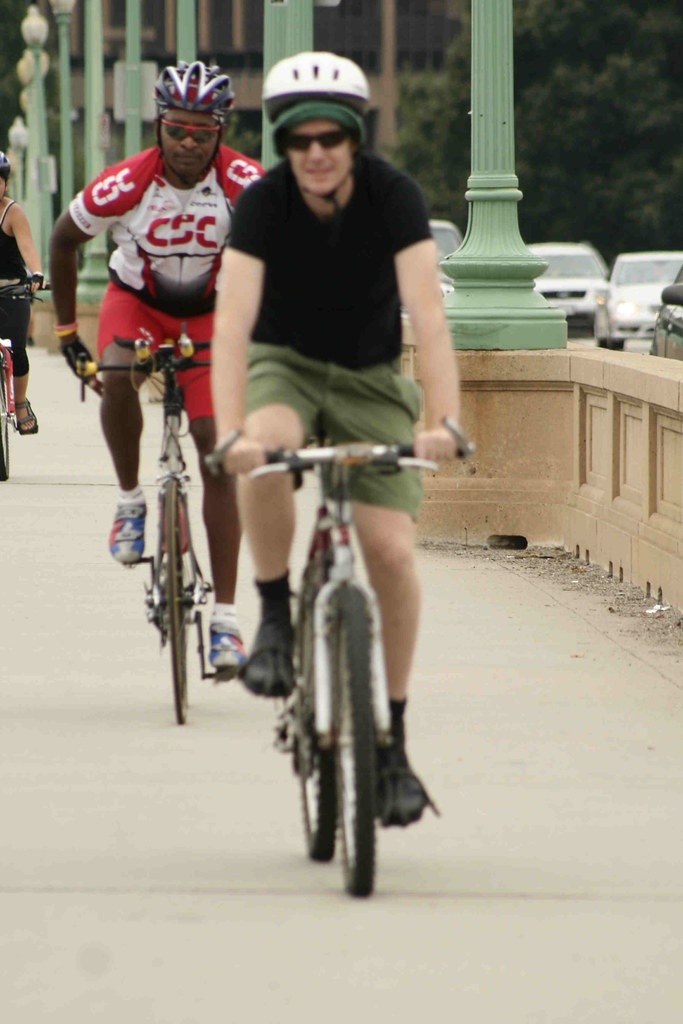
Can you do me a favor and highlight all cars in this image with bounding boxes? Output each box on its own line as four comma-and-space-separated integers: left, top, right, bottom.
590, 247, 683, 351
648, 262, 683, 362
525, 239, 610, 336
428, 217, 464, 300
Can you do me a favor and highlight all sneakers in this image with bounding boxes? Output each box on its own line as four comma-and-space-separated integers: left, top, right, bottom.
108, 496, 147, 561
374, 744, 440, 827
243, 621, 295, 695
209, 622, 247, 667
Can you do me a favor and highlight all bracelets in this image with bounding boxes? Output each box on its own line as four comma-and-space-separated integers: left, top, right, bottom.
54, 321, 78, 337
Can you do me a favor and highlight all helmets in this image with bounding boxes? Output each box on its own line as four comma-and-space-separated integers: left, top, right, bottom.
0, 151, 10, 179
260, 50, 371, 124
152, 60, 235, 124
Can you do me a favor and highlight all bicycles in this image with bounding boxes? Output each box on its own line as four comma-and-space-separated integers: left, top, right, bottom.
0, 276, 52, 485
203, 413, 476, 903
71, 322, 248, 728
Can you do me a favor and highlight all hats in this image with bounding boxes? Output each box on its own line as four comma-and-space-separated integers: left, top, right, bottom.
272, 99, 368, 160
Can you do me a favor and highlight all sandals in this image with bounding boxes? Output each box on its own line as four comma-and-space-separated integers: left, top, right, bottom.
15, 398, 37, 434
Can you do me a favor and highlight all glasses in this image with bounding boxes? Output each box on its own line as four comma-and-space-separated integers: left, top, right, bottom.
281, 128, 349, 151
160, 119, 221, 143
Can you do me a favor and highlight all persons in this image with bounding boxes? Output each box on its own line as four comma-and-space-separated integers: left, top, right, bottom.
0, 151, 47, 430
210, 51, 460, 828
48, 60, 265, 667
